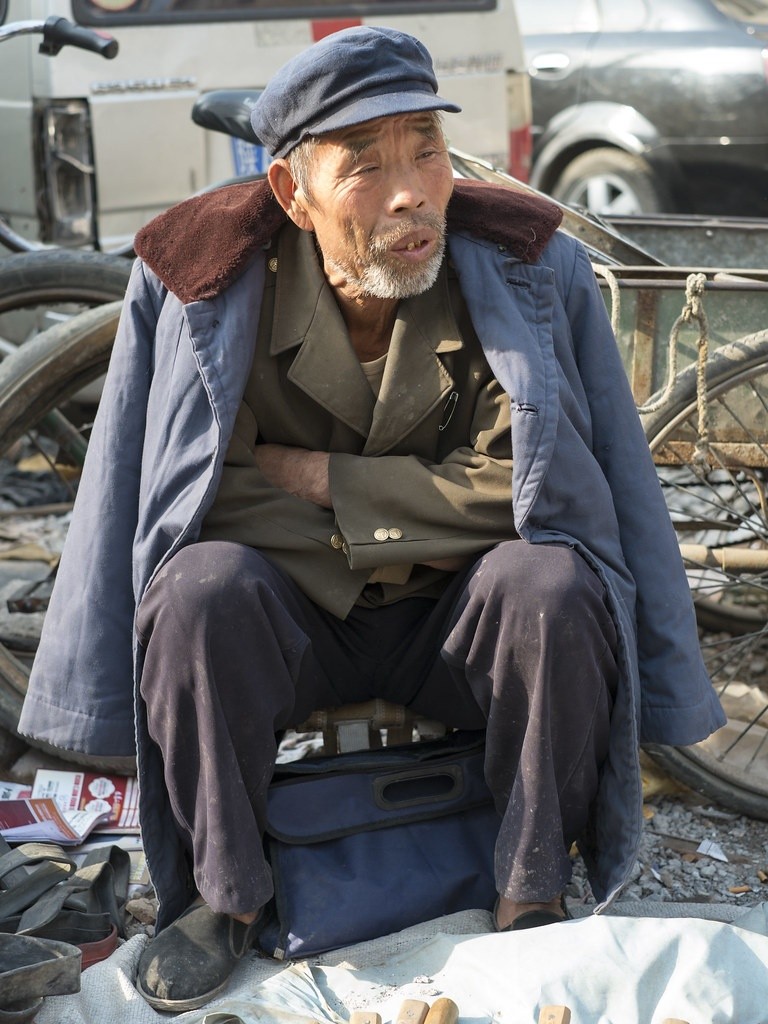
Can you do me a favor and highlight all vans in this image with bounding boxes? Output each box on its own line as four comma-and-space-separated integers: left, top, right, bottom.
0, 0, 535, 269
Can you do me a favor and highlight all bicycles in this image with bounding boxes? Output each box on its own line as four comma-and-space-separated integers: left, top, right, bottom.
0, 15, 768, 826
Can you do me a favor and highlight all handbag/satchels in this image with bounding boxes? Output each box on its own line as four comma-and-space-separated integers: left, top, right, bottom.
248, 729, 497, 959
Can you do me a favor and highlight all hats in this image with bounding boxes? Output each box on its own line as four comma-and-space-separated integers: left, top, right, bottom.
249, 26, 463, 167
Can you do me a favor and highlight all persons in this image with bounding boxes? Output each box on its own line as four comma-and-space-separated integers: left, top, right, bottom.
128, 24, 629, 1015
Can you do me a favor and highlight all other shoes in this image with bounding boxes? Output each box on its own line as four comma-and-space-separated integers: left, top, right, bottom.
490, 889, 571, 935
135, 897, 271, 1011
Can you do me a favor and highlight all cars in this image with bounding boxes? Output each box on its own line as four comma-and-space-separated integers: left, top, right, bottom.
517, 1, 768, 218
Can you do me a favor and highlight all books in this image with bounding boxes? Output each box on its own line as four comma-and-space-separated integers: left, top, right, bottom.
0, 769, 149, 885
